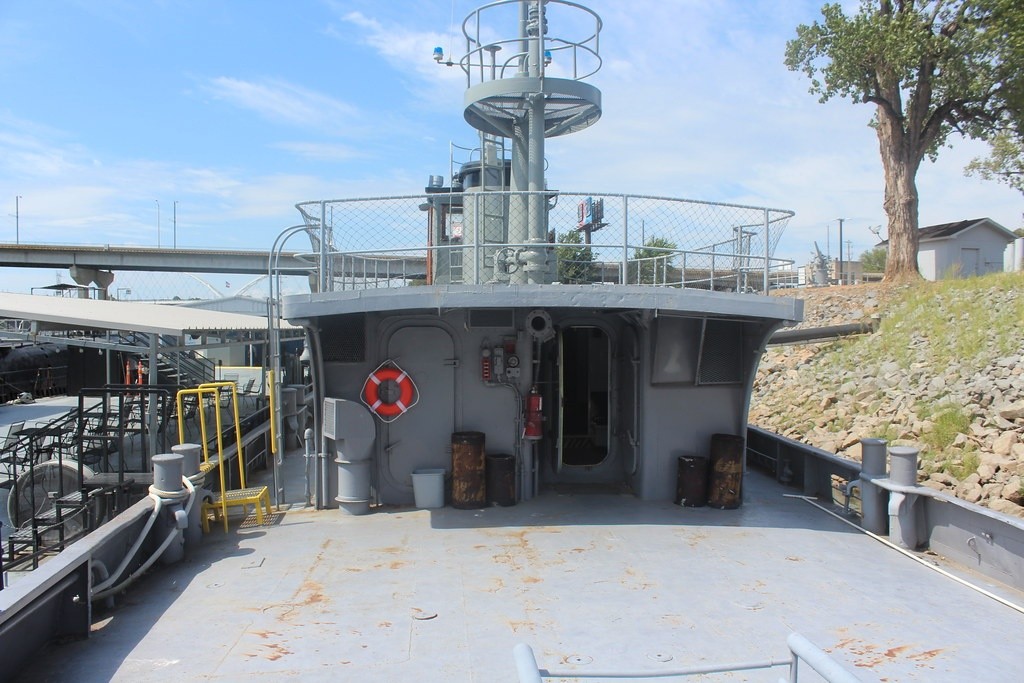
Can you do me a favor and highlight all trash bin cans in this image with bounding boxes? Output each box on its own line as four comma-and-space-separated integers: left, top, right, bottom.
412, 468, 447, 510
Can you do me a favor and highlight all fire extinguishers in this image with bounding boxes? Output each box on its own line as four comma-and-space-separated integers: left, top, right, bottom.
521, 383, 544, 440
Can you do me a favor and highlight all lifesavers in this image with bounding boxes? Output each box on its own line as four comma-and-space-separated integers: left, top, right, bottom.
364, 368, 414, 417
